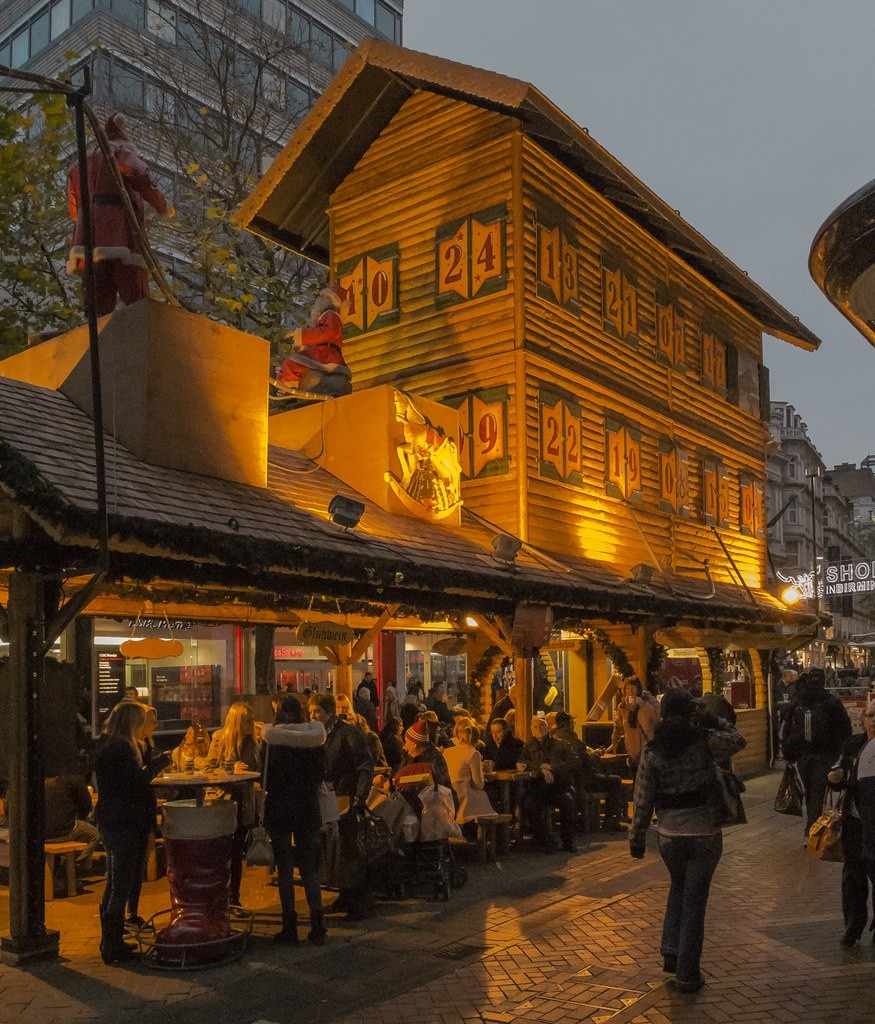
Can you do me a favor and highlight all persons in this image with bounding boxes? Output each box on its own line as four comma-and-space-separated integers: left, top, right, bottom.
603, 678, 660, 823
203, 702, 258, 917
486, 685, 515, 724
66, 114, 173, 315
46, 774, 100, 875
546, 713, 558, 735
94, 687, 172, 965
170, 724, 210, 773
768, 663, 850, 848
442, 726, 496, 861
481, 718, 525, 823
518, 716, 576, 852
276, 287, 354, 398
701, 692, 736, 725
827, 700, 875, 948
255, 692, 285, 732
336, 671, 485, 890
308, 691, 375, 894
552, 712, 628, 832
629, 687, 747, 993
257, 695, 328, 947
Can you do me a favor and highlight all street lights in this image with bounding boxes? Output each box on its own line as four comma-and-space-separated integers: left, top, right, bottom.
805, 466, 819, 616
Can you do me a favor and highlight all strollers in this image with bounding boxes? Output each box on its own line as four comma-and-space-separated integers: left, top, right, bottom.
384, 762, 467, 901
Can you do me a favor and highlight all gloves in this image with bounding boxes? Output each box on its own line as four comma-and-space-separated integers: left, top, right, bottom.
631, 846, 645, 858
692, 699, 719, 728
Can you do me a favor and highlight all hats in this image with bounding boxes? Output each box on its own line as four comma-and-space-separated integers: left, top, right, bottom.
555, 711, 577, 729
405, 720, 430, 744
660, 688, 697, 718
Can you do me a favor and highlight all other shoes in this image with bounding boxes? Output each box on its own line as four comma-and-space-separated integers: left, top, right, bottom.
274, 927, 299, 941
230, 901, 248, 917
675, 971, 705, 989
308, 927, 328, 944
322, 894, 354, 913
842, 917, 865, 946
343, 900, 377, 921
664, 956, 678, 973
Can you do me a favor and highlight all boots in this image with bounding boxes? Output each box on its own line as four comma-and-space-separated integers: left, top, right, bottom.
99, 905, 138, 953
101, 914, 142, 964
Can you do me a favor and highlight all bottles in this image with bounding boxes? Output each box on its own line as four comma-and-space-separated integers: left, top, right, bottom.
225, 758, 235, 774
185, 759, 195, 774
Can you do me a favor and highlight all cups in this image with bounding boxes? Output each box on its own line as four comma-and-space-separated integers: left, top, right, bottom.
537, 711, 545, 716
629, 697, 639, 705
516, 762, 528, 771
483, 760, 494, 772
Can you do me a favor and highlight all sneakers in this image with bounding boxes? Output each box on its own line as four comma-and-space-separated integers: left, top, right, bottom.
123, 928, 134, 940
123, 915, 154, 933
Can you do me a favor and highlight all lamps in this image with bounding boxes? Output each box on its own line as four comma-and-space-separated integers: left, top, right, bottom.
491, 532, 522, 563
629, 563, 656, 584
327, 494, 366, 531
778, 582, 802, 608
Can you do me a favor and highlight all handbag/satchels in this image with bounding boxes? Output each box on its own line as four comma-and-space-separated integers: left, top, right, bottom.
366, 773, 410, 837
319, 790, 341, 823
774, 763, 804, 817
418, 784, 466, 842
807, 785, 845, 863
348, 802, 392, 856
247, 827, 275, 866
703, 729, 748, 829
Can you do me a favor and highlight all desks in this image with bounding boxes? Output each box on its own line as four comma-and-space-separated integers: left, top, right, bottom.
134, 769, 260, 973
600, 753, 628, 775
374, 766, 392, 776
89, 792, 166, 881
483, 769, 540, 847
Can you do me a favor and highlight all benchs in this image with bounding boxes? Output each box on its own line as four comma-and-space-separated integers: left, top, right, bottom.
0, 839, 89, 902
587, 791, 610, 833
464, 814, 513, 863
